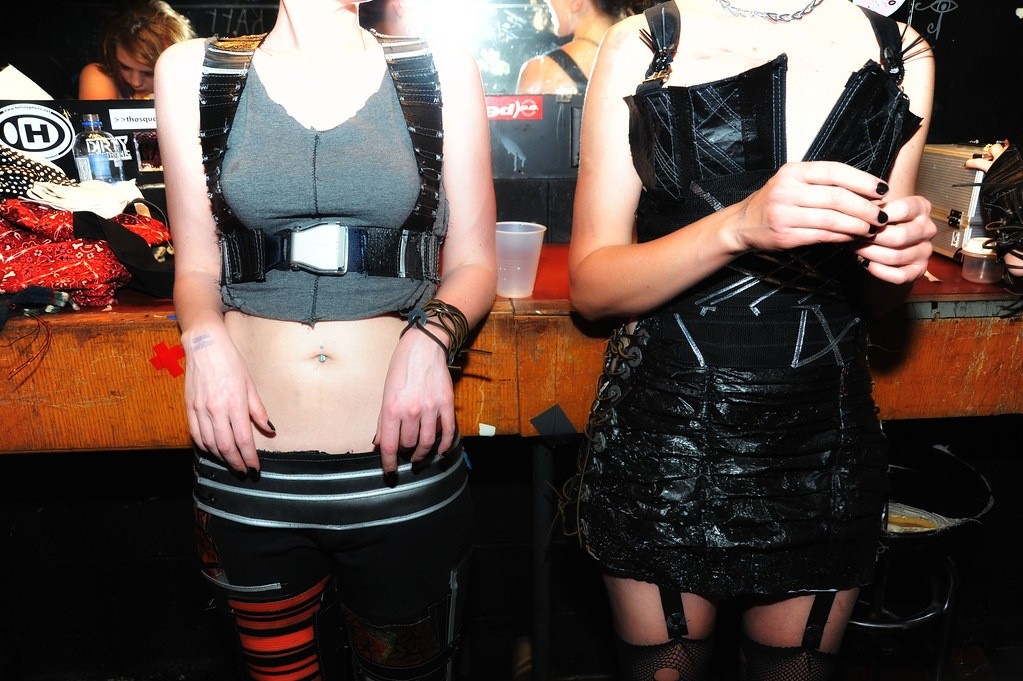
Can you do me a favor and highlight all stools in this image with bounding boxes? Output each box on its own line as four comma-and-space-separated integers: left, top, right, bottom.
830, 444, 995, 680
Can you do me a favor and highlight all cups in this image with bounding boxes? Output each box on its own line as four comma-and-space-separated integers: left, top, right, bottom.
496, 222, 546, 298
961, 250, 1000, 283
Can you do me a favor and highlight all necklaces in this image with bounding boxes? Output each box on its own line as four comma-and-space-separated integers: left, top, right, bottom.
718, 0, 823, 22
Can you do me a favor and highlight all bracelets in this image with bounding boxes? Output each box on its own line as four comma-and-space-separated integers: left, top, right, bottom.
401, 298, 494, 370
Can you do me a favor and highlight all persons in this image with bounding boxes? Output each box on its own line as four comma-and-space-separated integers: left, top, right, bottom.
515, 0, 636, 93
359, 0, 407, 36
153, 0, 494, 680
80, 0, 198, 100
980, 143, 1023, 276
569, 0, 938, 681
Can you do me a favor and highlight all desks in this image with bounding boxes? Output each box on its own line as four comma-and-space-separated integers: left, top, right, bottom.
1, 241, 1023, 453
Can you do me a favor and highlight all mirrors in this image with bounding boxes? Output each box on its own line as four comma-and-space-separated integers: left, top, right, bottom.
0, 0, 1023, 100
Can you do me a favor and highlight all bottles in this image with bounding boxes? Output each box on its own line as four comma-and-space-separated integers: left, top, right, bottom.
74, 114, 125, 186
